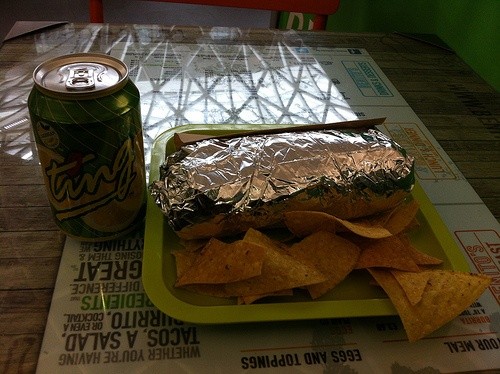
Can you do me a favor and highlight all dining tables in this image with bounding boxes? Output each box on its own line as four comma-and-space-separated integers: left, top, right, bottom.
1, 21, 500, 374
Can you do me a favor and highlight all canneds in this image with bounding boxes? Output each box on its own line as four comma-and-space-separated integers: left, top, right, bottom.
27, 51, 149, 243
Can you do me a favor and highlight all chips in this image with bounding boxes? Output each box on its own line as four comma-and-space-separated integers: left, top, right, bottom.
167, 200, 492, 343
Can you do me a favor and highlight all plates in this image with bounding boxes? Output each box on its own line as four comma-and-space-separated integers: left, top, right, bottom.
142, 124, 471, 324
